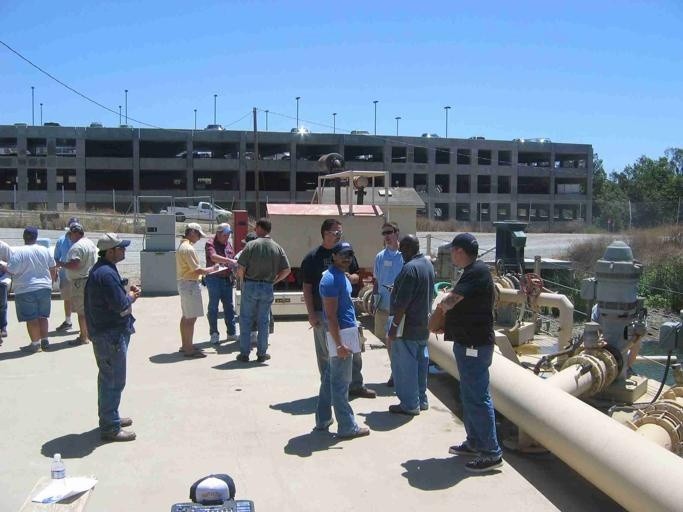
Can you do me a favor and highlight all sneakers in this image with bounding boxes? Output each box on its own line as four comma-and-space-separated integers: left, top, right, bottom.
0, 322, 91, 352
315, 373, 503, 472
108, 428, 136, 440
180, 330, 274, 361
119, 417, 132, 426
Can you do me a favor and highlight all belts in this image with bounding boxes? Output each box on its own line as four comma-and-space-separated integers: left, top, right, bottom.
245, 278, 272, 284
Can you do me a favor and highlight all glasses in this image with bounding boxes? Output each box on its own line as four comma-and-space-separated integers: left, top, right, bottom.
328, 230, 343, 237
381, 230, 396, 235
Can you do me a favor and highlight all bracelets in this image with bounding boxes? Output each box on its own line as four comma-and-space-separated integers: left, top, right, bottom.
392, 321, 399, 328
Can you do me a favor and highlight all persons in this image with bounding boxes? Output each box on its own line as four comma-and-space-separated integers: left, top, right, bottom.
0, 241, 16, 344
85, 230, 142, 443
311, 242, 370, 440
55, 223, 97, 344
177, 218, 292, 365
0, 226, 56, 354
373, 222, 406, 387
388, 235, 434, 415
430, 233, 505, 471
302, 218, 377, 399
53, 217, 80, 332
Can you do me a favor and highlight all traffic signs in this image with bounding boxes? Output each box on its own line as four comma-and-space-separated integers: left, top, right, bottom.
30, 85, 35, 126
40, 103, 44, 125
125, 90, 129, 124
119, 106, 123, 124
265, 110, 269, 131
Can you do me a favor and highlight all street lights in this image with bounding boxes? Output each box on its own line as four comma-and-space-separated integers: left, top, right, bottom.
296, 97, 301, 128
373, 101, 378, 135
214, 94, 217, 124
444, 107, 451, 138
333, 113, 337, 134
193, 109, 197, 129
395, 117, 402, 136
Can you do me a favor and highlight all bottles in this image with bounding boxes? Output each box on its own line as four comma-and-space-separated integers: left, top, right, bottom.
51, 453, 66, 500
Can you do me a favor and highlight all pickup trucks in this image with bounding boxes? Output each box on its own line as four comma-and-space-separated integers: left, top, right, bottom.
165, 202, 231, 223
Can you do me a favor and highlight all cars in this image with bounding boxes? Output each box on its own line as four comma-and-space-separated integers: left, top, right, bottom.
421, 184, 444, 193
55, 147, 76, 156
536, 137, 551, 143
175, 149, 214, 158
274, 151, 292, 160
0, 146, 32, 156
391, 156, 407, 162
44, 122, 59, 126
422, 133, 439, 138
530, 160, 560, 168
14, 123, 28, 126
119, 124, 133, 128
226, 151, 259, 161
470, 137, 485, 139
513, 138, 529, 142
291, 127, 309, 133
351, 130, 370, 135
355, 152, 375, 161
91, 123, 103, 128
433, 207, 447, 217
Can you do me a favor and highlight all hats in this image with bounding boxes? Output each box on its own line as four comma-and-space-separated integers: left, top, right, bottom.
189, 475, 236, 503
445, 233, 478, 249
22, 218, 257, 251
332, 242, 353, 254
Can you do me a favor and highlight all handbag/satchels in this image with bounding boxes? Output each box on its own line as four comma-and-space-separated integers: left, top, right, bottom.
427, 307, 445, 336
